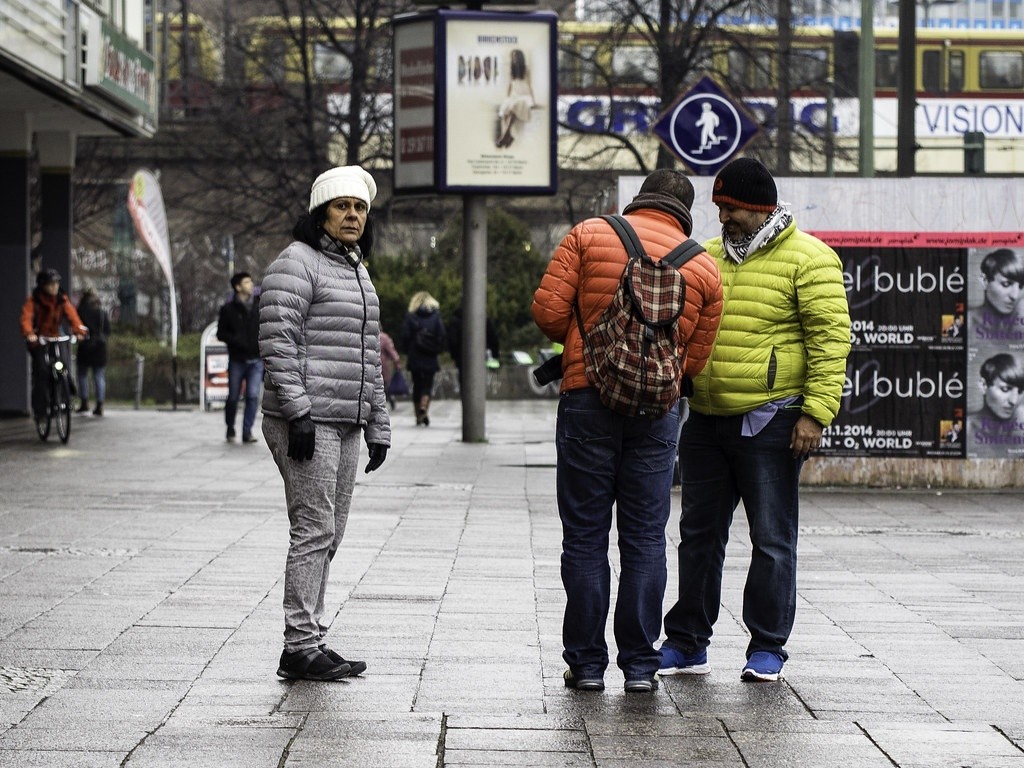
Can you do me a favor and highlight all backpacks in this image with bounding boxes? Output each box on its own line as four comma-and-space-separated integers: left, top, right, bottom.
574, 213, 706, 420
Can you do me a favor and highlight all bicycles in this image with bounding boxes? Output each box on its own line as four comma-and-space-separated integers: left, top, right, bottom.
25, 335, 90, 442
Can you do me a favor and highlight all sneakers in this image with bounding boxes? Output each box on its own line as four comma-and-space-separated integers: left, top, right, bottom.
624, 678, 658, 691
656, 644, 711, 676
326, 650, 367, 676
740, 652, 784, 681
563, 670, 604, 689
277, 649, 350, 680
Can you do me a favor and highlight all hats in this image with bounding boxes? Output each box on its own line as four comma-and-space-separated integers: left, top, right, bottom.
308, 166, 378, 215
712, 158, 778, 213
37, 269, 62, 286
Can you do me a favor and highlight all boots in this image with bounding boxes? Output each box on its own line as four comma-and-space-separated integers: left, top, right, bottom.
94, 402, 102, 415
415, 396, 431, 426
77, 399, 90, 412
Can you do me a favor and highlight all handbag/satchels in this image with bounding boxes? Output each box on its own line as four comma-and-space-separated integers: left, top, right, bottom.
386, 367, 410, 395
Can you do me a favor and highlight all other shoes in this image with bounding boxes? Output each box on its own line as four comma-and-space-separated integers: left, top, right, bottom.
244, 434, 257, 441
226, 426, 235, 436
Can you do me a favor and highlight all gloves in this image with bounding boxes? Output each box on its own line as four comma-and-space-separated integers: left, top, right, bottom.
286, 411, 315, 461
365, 442, 387, 474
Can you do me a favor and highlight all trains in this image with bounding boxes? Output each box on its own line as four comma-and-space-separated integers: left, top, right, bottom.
146, 17, 1024, 138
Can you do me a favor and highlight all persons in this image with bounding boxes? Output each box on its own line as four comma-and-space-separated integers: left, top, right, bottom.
216, 272, 264, 444
74, 285, 112, 415
497, 51, 537, 148
405, 292, 446, 427
22, 268, 86, 420
373, 320, 401, 412
258, 166, 390, 682
655, 158, 851, 683
968, 248, 1024, 443
531, 168, 724, 693
